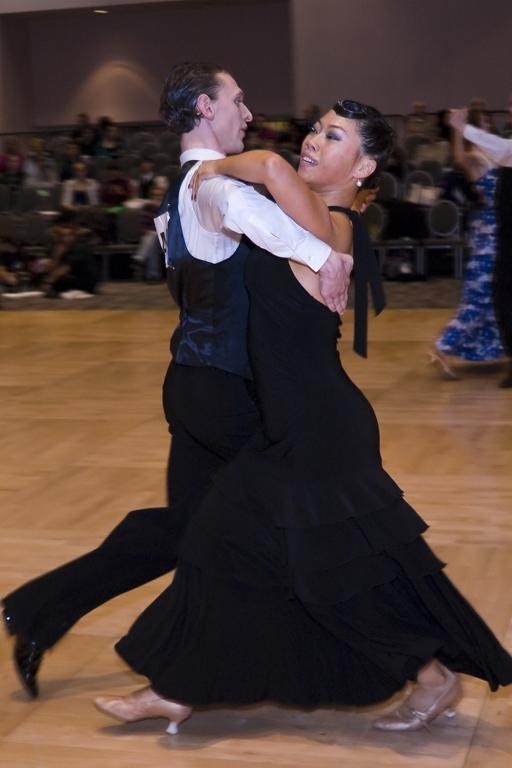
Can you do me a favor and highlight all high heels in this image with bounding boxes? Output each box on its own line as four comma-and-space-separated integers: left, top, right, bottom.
369, 670, 465, 732
425, 346, 457, 381
89, 685, 193, 736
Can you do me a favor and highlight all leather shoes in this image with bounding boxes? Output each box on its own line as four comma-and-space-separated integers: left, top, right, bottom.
1, 602, 43, 698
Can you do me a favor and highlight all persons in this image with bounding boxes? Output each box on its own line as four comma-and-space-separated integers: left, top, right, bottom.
238, 108, 511, 284
428, 108, 512, 386
92, 98, 511, 736
0, 60, 354, 693
0, 107, 190, 301
